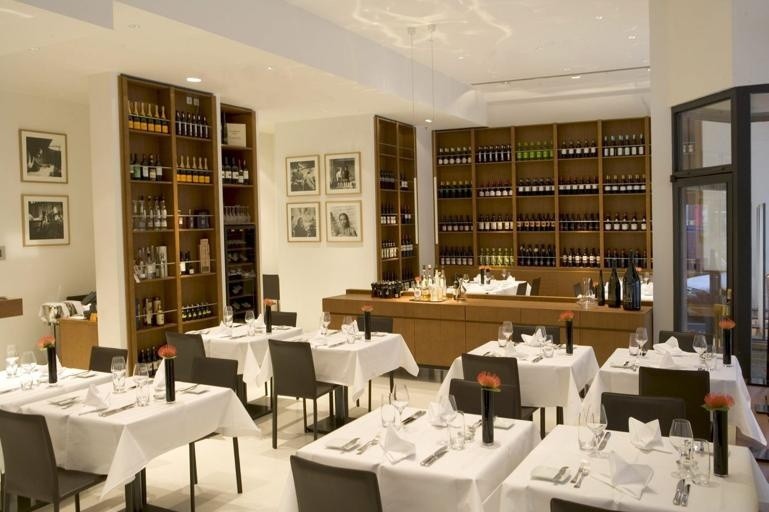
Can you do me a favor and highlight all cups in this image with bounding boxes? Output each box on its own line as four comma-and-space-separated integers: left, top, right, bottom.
463, 274, 469, 283
690, 439, 710, 487
224, 311, 233, 327
244, 311, 255, 335
635, 327, 648, 353
503, 321, 513, 342
321, 312, 331, 333
692, 335, 707, 357
586, 404, 608, 458
544, 335, 554, 358
577, 411, 595, 451
21, 351, 37, 374
223, 306, 232, 313
439, 395, 456, 449
502, 269, 507, 280
136, 382, 150, 407
628, 333, 639, 356
447, 411, 465, 451
392, 383, 409, 422
670, 418, 694, 478
535, 326, 546, 354
381, 393, 395, 427
132, 363, 149, 392
497, 327, 506, 348
111, 357, 127, 393
318, 317, 327, 334
6, 353, 18, 378
6, 345, 19, 365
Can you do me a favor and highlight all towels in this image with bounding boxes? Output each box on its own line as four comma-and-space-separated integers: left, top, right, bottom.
531, 465, 571, 483
652, 336, 682, 356
79, 382, 106, 412
381, 426, 415, 465
520, 327, 551, 347
608, 450, 654, 501
609, 358, 634, 368
627, 417, 663, 452
326, 436, 360, 450
655, 351, 675, 368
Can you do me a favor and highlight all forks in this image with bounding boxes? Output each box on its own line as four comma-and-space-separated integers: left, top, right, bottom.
357, 435, 381, 451
571, 461, 584, 484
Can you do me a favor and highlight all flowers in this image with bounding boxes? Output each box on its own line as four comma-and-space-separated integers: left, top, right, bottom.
719, 319, 736, 329
263, 299, 276, 307
560, 311, 575, 322
158, 344, 176, 358
700, 393, 734, 411
38, 336, 55, 348
361, 304, 374, 312
477, 370, 502, 393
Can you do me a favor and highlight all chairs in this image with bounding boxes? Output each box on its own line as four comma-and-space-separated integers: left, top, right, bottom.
164, 331, 248, 410
638, 366, 711, 440
1, 410, 109, 511
599, 391, 687, 437
448, 380, 517, 420
268, 339, 346, 451
264, 310, 297, 327
263, 275, 280, 312
658, 330, 707, 353
460, 353, 539, 420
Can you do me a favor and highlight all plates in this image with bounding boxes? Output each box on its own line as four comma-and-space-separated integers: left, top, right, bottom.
410, 296, 447, 302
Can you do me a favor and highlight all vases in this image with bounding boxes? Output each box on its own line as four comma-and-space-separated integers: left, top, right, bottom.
364, 313, 371, 340
265, 306, 272, 333
48, 347, 57, 383
165, 359, 175, 401
712, 411, 729, 477
565, 322, 574, 354
481, 389, 495, 444
723, 330, 732, 365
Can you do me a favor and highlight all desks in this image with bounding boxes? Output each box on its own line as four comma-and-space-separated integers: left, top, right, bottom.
687, 303, 733, 354
322, 289, 466, 371
465, 294, 655, 367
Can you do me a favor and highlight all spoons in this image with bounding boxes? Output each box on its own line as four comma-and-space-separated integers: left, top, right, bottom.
574, 468, 589, 489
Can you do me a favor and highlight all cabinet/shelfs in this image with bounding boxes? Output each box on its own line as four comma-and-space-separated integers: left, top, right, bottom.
55, 318, 98, 369
374, 115, 420, 293
219, 102, 262, 324
431, 116, 652, 301
117, 74, 224, 379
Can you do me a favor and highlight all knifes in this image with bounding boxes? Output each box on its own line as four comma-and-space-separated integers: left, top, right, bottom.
672, 477, 685, 505
681, 484, 690, 506
598, 432, 610, 450
98, 402, 136, 417
402, 410, 426, 425
552, 467, 568, 482
419, 445, 448, 467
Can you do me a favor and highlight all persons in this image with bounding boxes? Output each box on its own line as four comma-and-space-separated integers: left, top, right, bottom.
336, 213, 357, 237
335, 166, 342, 186
343, 165, 350, 182
294, 217, 308, 238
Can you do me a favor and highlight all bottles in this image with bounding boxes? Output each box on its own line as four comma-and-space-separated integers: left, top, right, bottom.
184, 253, 188, 274
453, 215, 459, 231
498, 215, 503, 231
401, 233, 413, 257
523, 213, 529, 231
450, 180, 458, 198
449, 147, 455, 164
156, 153, 163, 181
152, 346, 157, 375
192, 114, 197, 137
198, 157, 204, 183
196, 304, 202, 319
567, 140, 575, 158
588, 213, 595, 230
575, 213, 581, 231
188, 251, 194, 274
530, 141, 536, 160
546, 213, 551, 231
243, 159, 250, 185
500, 144, 505, 161
589, 248, 596, 267
467, 145, 472, 164
620, 175, 626, 193
445, 246, 450, 265
568, 248, 575, 267
562, 247, 568, 266
156, 354, 162, 369
401, 204, 411, 224
139, 195, 145, 227
609, 136, 616, 156
582, 247, 589, 267
638, 133, 645, 155
624, 135, 630, 155
579, 176, 584, 194
491, 215, 497, 230
552, 244, 556, 267
596, 249, 600, 267
154, 196, 160, 228
187, 112, 193, 137
490, 184, 495, 196
502, 183, 508, 196
585, 175, 591, 194
133, 101, 140, 130
503, 215, 509, 231
616, 135, 624, 156
485, 214, 490, 231
627, 248, 634, 265
561, 140, 567, 158
232, 157, 238, 183
626, 175, 633, 193
631, 211, 637, 231
470, 217, 473, 231
559, 213, 564, 231
504, 248, 509, 264
552, 177, 555, 195
176, 163, 180, 183
510, 215, 513, 230
147, 104, 154, 132
545, 175, 551, 195
490, 248, 497, 264
569, 213, 575, 231
464, 179, 470, 197
413, 264, 446, 301
204, 157, 210, 183
484, 185, 489, 197
439, 181, 445, 198
441, 216, 447, 231
496, 248, 503, 264
613, 212, 620, 231
517, 142, 522, 159
176, 111, 182, 135
633, 175, 641, 193
575, 248, 582, 267
518, 178, 525, 196
477, 214, 484, 231
136, 244, 165, 279
611, 175, 619, 193
592, 175, 598, 194
485, 248, 490, 264
509, 184, 512, 196
495, 184, 501, 196
180, 248, 186, 275
582, 213, 588, 231
380, 170, 395, 189
382, 240, 397, 259
478, 146, 483, 162
641, 216, 647, 231
455, 146, 461, 164
506, 144, 511, 160
462, 247, 467, 265
575, 141, 582, 157
447, 216, 452, 231
480, 248, 485, 264
527, 244, 533, 265
154, 105, 161, 132
205, 302, 211, 317
539, 244, 546, 266
523, 142, 529, 160
161, 105, 168, 134
186, 155, 192, 182
595, 213, 599, 230
573, 176, 578, 194
238, 159, 243, 171
222, 164, 225, 184
583, 140, 590, 157
179, 155, 186, 182
146, 348, 152, 377
623, 263, 641, 310
456, 247, 461, 265
450, 247, 456, 264
182, 306, 187, 321
186, 305, 192, 321
443, 147, 450, 165
130, 153, 134, 179
459, 215, 464, 231
132, 200, 139, 229
551, 213, 555, 231
181, 112, 187, 136
604, 175, 611, 193
519, 243, 525, 265
141, 154, 149, 180
464, 215, 470, 231
147, 195, 154, 229
546, 244, 552, 266
381, 200, 396, 224
631, 134, 638, 155
564, 213, 569, 231
525, 177, 531, 196
156, 304, 165, 326
606, 248, 610, 267
138, 348, 147, 375
543, 139, 549, 159
541, 213, 546, 231
444, 181, 451, 198
539, 177, 545, 195
634, 249, 640, 267
517, 216, 522, 230
479, 186, 484, 197
488, 145, 494, 161
494, 145, 499, 161
458, 180, 464, 197
201, 303, 206, 318
185, 209, 194, 228
160, 193, 168, 227
642, 249, 647, 268
536, 213, 541, 231
621, 249, 627, 268
613, 248, 618, 267
603, 136, 609, 156
202, 115, 208, 139
467, 247, 473, 266
565, 176, 572, 195
520, 213, 523, 231
590, 140, 597, 157
192, 156, 198, 183
149, 153, 156, 181
622, 212, 629, 231
605, 211, 612, 231
140, 102, 147, 131
225, 156, 232, 184
190, 304, 197, 320
549, 139, 553, 159
440, 246, 445, 264
530, 213, 535, 231
133, 153, 141, 180
536, 140, 542, 159
558, 176, 565, 195
510, 248, 514, 265
438, 146, 443, 165
608, 268, 620, 307
461, 145, 468, 163
483, 145, 488, 162
128, 100, 133, 129
371, 280, 403, 298
178, 209, 183, 228
197, 114, 203, 137
641, 174, 646, 193
597, 269, 605, 306
533, 244, 540, 266
400, 175, 408, 190
470, 180, 472, 196
532, 177, 538, 195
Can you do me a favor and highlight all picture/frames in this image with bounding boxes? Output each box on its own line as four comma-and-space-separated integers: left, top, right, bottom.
286, 202, 321, 243
325, 200, 363, 243
19, 129, 68, 185
285, 155, 321, 197
20, 194, 71, 247
324, 152, 362, 195
